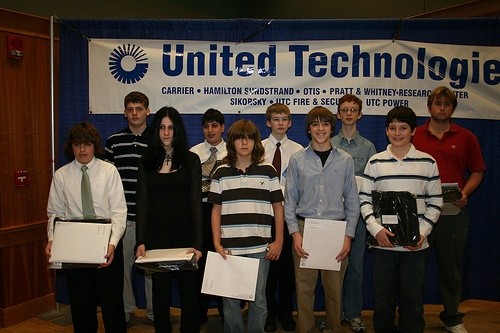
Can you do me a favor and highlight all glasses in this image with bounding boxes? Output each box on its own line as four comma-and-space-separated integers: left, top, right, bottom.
339, 108, 359, 114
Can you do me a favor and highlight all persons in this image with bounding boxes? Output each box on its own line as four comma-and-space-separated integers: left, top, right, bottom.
103, 90, 154, 325
316, 94, 377, 333
410, 86, 486, 333
258, 101, 305, 333
188, 108, 229, 324
132, 106, 208, 333
207, 120, 284, 333
358, 106, 444, 333
44, 122, 128, 333
285, 107, 361, 333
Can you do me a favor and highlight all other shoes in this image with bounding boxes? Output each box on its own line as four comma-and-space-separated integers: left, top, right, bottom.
264, 316, 277, 332
318, 316, 345, 330
146, 312, 154, 321
126, 312, 131, 322
277, 313, 296, 332
344, 317, 367, 333
443, 322, 467, 333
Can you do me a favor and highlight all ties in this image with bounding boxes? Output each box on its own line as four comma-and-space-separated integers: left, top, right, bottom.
272, 142, 281, 183
202, 147, 218, 189
81, 165, 96, 220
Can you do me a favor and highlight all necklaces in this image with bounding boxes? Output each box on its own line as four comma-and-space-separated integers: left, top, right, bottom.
165, 154, 172, 165
